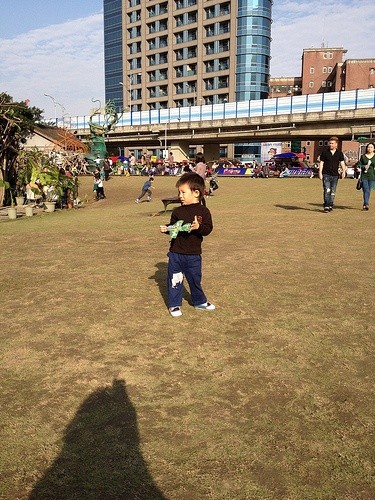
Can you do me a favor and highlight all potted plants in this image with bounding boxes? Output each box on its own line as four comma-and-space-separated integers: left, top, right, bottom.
24, 159, 47, 216
15, 150, 27, 205
0, 179, 17, 220
39, 164, 81, 212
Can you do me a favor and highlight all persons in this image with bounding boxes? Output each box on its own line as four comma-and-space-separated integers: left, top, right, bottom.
58, 150, 375, 210
158, 173, 217, 317
318, 136, 346, 213
134, 177, 154, 204
356, 143, 375, 210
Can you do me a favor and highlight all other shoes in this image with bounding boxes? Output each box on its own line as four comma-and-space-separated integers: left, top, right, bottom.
356, 178, 362, 190
135, 200, 138, 204
196, 302, 215, 310
324, 206, 329, 212
363, 205, 369, 210
329, 206, 332, 210
169, 306, 182, 316
147, 198, 151, 201
